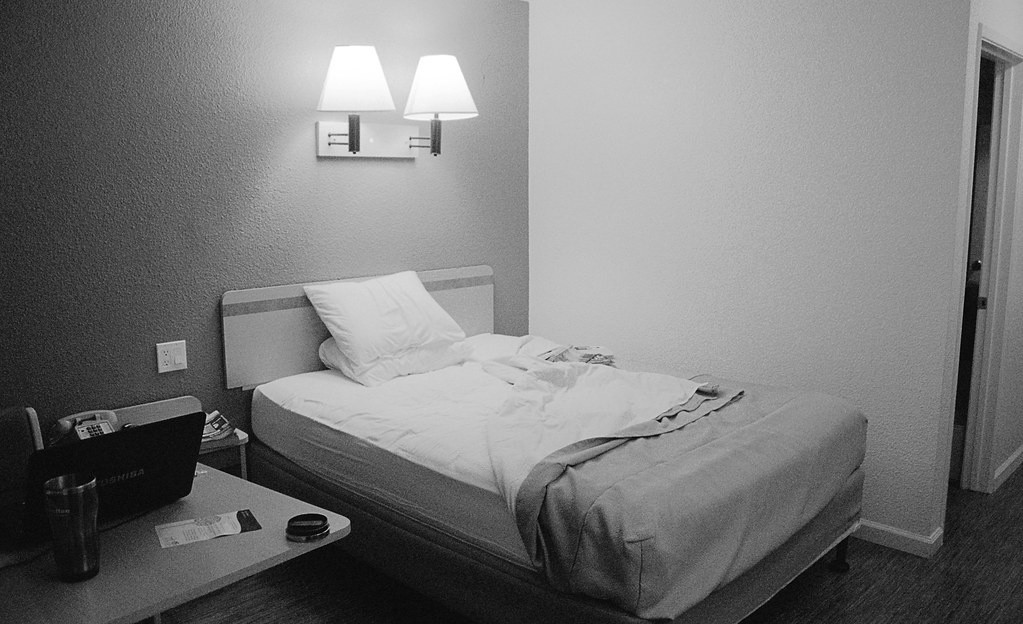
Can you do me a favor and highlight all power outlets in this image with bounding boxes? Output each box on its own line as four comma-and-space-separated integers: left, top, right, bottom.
156, 339, 188, 373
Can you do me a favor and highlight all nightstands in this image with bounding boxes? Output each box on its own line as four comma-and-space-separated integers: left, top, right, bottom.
198, 412, 250, 483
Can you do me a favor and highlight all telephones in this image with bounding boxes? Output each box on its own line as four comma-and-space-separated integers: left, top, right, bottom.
58, 410, 119, 442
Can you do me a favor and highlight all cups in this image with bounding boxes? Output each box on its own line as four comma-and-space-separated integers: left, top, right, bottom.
42, 472, 99, 582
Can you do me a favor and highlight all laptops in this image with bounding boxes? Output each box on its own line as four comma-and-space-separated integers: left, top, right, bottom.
33, 413, 208, 538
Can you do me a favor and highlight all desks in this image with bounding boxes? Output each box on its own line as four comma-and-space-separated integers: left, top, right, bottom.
0, 462, 350, 624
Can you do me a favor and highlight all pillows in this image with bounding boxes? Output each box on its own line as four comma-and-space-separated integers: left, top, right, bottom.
318, 335, 466, 386
303, 271, 466, 365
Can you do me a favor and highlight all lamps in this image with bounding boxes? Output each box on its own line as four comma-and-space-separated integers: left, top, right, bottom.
317, 44, 398, 154
403, 55, 478, 156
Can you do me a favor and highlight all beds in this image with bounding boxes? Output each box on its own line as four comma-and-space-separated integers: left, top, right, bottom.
221, 263, 868, 624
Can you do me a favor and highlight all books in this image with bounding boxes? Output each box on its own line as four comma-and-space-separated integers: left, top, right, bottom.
200, 409, 240, 452
